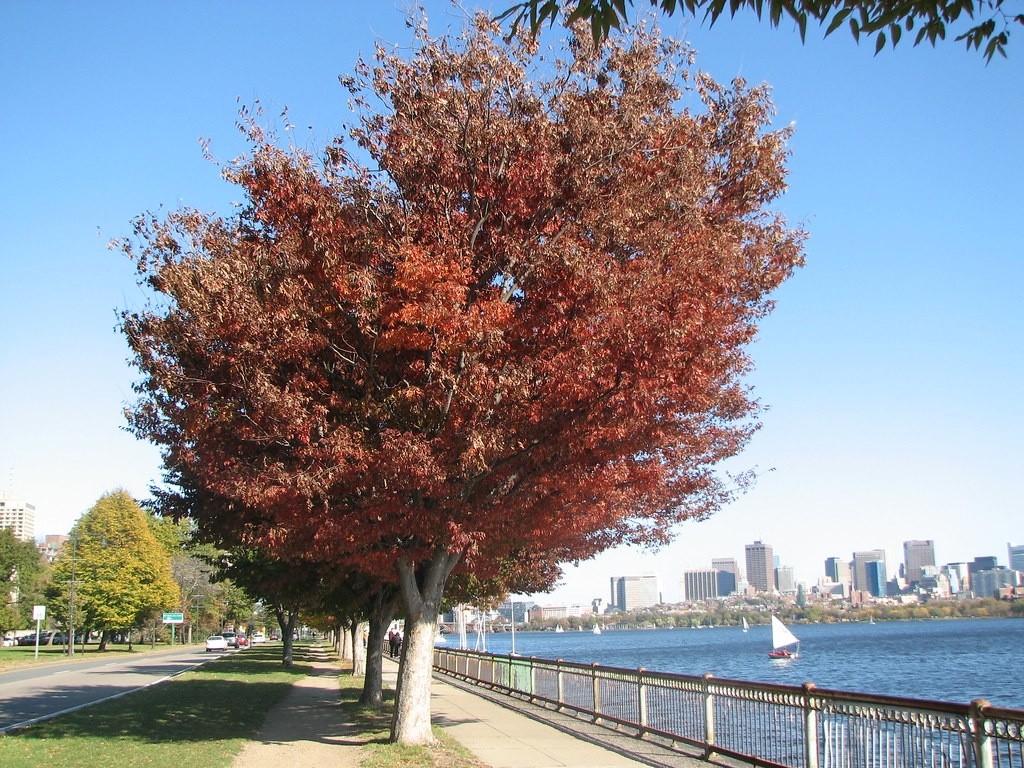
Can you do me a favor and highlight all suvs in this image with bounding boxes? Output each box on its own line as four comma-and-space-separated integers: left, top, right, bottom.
220, 631, 240, 649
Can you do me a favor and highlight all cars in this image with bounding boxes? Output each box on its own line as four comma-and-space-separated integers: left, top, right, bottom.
236, 634, 248, 646
251, 634, 266, 643
269, 634, 278, 641
17, 630, 81, 645
292, 633, 298, 641
205, 635, 230, 652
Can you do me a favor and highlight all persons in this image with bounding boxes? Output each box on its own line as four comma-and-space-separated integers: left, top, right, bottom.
389, 628, 395, 658
392, 632, 402, 656
363, 630, 369, 648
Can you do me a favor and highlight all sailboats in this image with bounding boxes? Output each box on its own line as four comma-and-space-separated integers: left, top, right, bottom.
768, 614, 801, 659
